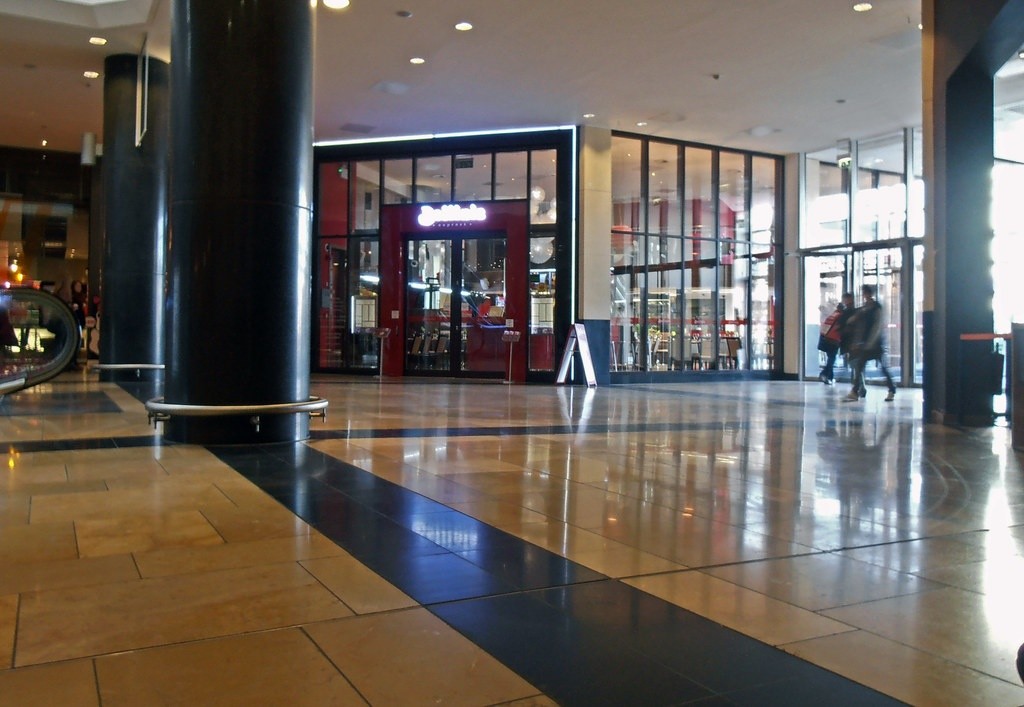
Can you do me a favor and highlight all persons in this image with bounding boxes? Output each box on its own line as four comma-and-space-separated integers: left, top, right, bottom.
38, 280, 100, 372
818, 285, 896, 402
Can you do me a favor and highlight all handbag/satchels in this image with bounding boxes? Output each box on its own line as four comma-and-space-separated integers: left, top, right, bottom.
818, 333, 828, 353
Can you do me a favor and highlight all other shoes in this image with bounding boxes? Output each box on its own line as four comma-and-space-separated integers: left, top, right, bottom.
842, 393, 859, 401
885, 392, 894, 401
819, 374, 832, 384
828, 377, 836, 384
74, 364, 84, 371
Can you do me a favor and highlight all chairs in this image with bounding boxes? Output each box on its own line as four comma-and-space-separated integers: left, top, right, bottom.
655, 337, 743, 371
406, 334, 448, 371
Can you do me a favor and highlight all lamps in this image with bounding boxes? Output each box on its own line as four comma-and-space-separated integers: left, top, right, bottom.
80, 132, 103, 166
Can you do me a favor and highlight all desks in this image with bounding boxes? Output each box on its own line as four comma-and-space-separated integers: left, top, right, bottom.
655, 339, 669, 367
689, 341, 704, 369
408, 338, 467, 369
